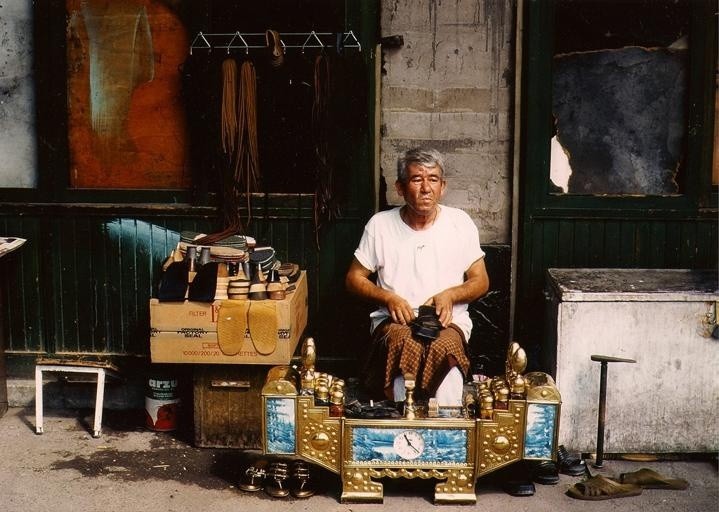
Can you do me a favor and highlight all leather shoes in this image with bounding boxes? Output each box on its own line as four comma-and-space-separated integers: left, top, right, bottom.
557, 445, 586, 476
525, 460, 560, 484
500, 462, 534, 497
237, 459, 317, 498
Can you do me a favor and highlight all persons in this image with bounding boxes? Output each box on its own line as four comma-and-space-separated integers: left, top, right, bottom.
343, 144, 492, 410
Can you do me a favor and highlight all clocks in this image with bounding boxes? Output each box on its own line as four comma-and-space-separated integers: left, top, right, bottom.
393, 429, 426, 460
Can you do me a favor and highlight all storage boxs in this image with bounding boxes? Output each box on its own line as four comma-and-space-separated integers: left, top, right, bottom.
150, 269, 310, 365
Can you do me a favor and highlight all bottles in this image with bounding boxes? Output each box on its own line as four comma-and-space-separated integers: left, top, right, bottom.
185, 245, 212, 283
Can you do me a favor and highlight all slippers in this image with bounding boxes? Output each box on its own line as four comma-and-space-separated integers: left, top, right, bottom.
612, 469, 690, 490
568, 473, 643, 501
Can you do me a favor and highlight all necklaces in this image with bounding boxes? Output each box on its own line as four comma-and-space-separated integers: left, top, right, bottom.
402, 205, 438, 226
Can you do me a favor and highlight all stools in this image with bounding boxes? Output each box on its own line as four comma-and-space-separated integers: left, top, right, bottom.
31, 357, 125, 437
